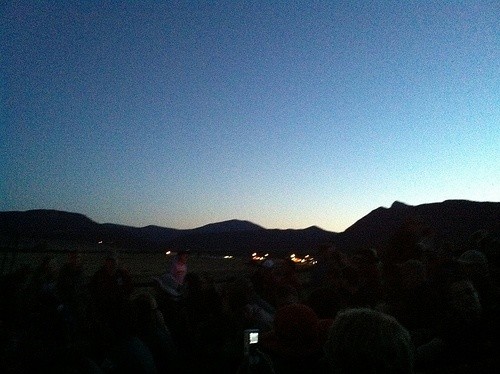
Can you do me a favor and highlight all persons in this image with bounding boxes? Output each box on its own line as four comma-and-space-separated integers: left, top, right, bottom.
0, 223, 500, 374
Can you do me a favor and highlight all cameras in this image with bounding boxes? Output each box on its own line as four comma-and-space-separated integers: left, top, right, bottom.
243, 329, 262, 356
